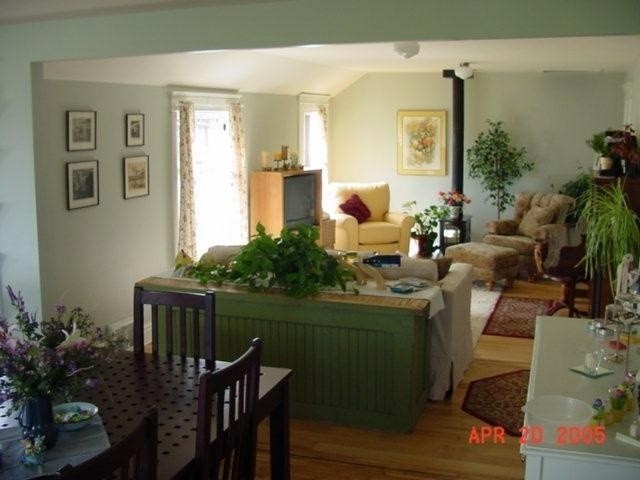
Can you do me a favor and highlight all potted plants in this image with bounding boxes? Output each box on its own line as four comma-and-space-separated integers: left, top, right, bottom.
403, 201, 450, 257
188, 221, 362, 297
587, 134, 616, 170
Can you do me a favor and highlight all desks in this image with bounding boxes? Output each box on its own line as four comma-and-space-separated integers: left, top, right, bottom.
135, 263, 439, 434
519, 316, 640, 480
198, 245, 472, 403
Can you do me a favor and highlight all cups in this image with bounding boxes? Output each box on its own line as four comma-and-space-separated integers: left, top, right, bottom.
585, 348, 604, 373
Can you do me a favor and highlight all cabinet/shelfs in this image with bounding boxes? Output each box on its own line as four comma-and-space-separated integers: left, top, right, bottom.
590, 167, 639, 318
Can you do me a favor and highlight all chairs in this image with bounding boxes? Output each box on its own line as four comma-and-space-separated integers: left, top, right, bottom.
57, 406, 158, 480
534, 223, 602, 317
133, 285, 215, 360
192, 337, 262, 480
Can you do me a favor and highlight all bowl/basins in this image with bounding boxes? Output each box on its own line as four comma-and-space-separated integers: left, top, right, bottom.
520, 396, 599, 445
53, 402, 97, 431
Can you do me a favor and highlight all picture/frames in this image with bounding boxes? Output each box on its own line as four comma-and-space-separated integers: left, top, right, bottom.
123, 156, 149, 200
67, 161, 102, 210
125, 113, 146, 147
68, 108, 97, 150
396, 109, 448, 177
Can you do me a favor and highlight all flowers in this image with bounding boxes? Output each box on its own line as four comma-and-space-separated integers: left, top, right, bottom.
438, 190, 472, 206
1, 285, 128, 418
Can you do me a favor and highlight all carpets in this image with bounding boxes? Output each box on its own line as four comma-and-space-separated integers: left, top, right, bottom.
462, 369, 531, 436
470, 279, 504, 348
483, 297, 554, 339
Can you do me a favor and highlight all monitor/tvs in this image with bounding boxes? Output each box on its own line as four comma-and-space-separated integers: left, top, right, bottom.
284, 174, 316, 231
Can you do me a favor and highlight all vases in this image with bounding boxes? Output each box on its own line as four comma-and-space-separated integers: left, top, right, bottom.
451, 206, 463, 219
18, 395, 60, 451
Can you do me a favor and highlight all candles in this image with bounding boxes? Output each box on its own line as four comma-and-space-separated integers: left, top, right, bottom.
274, 152, 281, 159
281, 145, 288, 159
262, 150, 272, 168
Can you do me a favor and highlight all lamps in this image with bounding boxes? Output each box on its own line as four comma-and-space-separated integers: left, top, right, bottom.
455, 63, 474, 80
393, 42, 420, 58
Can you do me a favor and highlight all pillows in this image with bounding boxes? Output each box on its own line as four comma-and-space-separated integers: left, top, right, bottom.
341, 195, 370, 223
435, 258, 452, 280
519, 206, 555, 240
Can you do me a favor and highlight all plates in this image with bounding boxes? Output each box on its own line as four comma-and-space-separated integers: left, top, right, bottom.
570, 364, 616, 378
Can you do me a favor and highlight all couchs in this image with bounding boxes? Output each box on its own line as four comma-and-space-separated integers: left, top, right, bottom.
446, 241, 520, 291
485, 192, 575, 283
321, 182, 415, 257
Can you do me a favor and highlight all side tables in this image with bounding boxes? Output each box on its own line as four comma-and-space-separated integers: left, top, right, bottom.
440, 214, 472, 253
